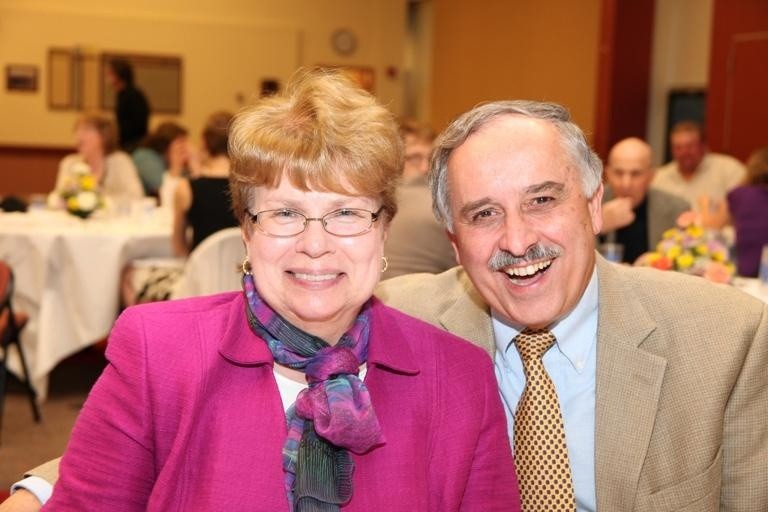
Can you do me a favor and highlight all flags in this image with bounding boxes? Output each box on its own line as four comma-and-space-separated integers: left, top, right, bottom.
69, 210, 92, 219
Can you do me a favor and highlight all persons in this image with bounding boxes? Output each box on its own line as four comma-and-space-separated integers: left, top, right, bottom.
35, 65, 522, 512
106, 53, 153, 155
650, 121, 748, 229
1, 100, 768, 511
384, 114, 461, 280
135, 121, 192, 200
45, 114, 146, 206
594, 137, 699, 268
726, 150, 767, 281
172, 111, 241, 260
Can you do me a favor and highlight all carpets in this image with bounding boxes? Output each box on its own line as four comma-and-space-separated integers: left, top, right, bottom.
513, 329, 576, 512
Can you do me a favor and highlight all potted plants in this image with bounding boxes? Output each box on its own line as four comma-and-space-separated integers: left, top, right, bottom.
101, 53, 182, 113
6, 65, 37, 91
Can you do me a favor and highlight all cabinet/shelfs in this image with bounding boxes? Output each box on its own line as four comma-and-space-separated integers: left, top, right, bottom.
600, 229, 624, 264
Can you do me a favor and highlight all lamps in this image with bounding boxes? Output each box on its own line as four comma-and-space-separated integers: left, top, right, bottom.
172, 227, 247, 301
0, 260, 41, 429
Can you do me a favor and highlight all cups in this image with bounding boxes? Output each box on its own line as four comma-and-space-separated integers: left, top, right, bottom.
243, 206, 384, 236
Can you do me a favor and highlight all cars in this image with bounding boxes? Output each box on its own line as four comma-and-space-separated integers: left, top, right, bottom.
131, 196, 157, 225
28, 195, 47, 215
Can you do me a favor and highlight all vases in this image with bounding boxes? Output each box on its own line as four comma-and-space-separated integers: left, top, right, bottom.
59, 161, 117, 211
632, 209, 736, 282
